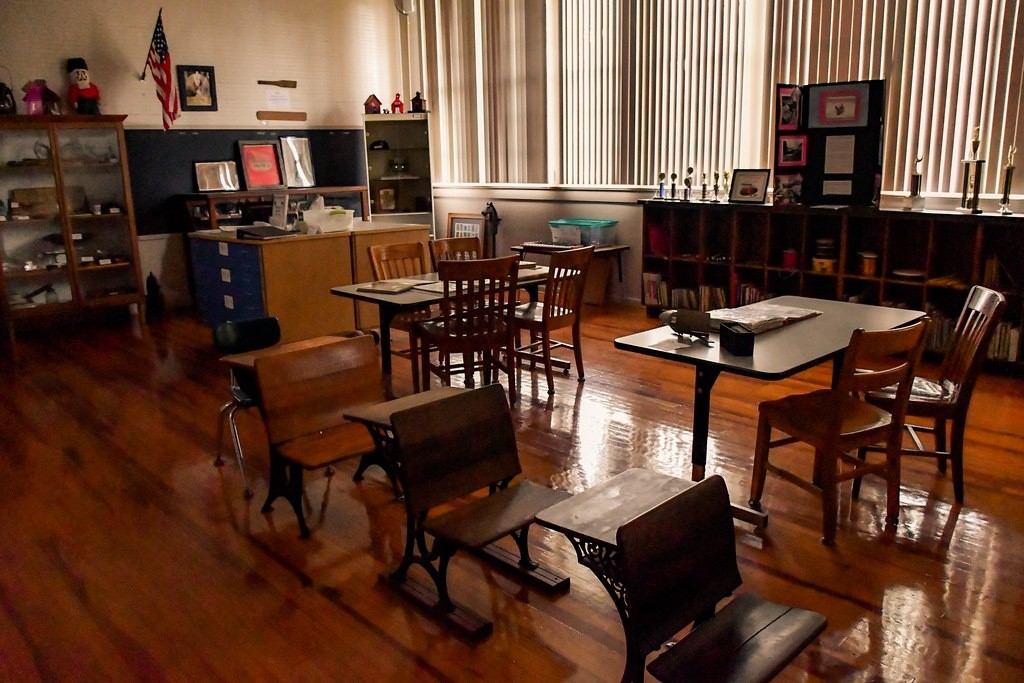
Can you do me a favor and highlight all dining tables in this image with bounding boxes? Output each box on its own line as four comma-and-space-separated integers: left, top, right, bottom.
613, 294, 926, 527
327, 263, 579, 393
220, 333, 350, 511
511, 242, 629, 299
345, 385, 476, 558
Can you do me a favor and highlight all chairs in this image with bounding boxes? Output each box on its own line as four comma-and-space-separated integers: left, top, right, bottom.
851, 285, 1005, 505
217, 317, 827, 683
747, 317, 932, 545
366, 236, 595, 407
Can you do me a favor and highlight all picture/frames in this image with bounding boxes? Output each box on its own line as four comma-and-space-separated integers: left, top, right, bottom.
238, 140, 285, 192
193, 160, 241, 193
279, 135, 316, 187
729, 168, 770, 202
446, 212, 486, 260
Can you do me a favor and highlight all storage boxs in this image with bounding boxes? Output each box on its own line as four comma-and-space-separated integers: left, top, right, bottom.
720, 323, 755, 356
548, 218, 617, 246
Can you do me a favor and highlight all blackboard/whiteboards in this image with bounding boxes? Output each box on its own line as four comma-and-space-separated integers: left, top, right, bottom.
123, 124, 370, 240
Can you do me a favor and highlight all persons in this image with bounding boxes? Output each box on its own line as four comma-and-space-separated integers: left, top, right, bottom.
791, 85, 801, 117
67, 58, 101, 114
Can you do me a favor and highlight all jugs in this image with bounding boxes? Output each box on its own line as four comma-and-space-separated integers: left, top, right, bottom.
0, 66, 17, 114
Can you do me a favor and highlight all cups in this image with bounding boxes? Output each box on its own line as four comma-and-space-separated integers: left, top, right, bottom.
783, 250, 800, 269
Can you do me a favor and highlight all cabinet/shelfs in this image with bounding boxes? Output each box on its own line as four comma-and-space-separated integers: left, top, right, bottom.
637, 198, 1024, 381
364, 110, 435, 241
0, 115, 150, 336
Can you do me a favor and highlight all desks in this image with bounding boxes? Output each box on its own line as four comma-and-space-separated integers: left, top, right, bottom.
183, 188, 430, 344
533, 467, 700, 683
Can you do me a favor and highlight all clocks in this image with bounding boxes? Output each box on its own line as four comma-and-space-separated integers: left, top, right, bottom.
176, 64, 218, 111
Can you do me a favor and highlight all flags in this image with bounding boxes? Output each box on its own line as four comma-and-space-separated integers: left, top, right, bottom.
147, 15, 182, 132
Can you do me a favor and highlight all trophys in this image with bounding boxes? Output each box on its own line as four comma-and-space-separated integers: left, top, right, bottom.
902, 153, 925, 211
665, 173, 680, 200
653, 173, 665, 199
710, 171, 720, 202
997, 145, 1016, 215
723, 171, 730, 202
687, 167, 696, 200
956, 126, 982, 214
679, 178, 690, 201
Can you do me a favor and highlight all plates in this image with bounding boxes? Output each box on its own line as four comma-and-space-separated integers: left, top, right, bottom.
893, 271, 924, 277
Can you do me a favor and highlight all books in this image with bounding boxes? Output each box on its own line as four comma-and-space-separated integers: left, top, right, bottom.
699, 284, 726, 312
925, 296, 959, 349
741, 283, 769, 305
643, 272, 668, 306
987, 321, 1024, 361
704, 302, 824, 334
699, 173, 710, 201
358, 278, 437, 294
672, 288, 699, 309
508, 260, 549, 281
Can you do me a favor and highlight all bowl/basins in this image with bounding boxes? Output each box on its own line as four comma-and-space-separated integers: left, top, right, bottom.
812, 256, 837, 273
